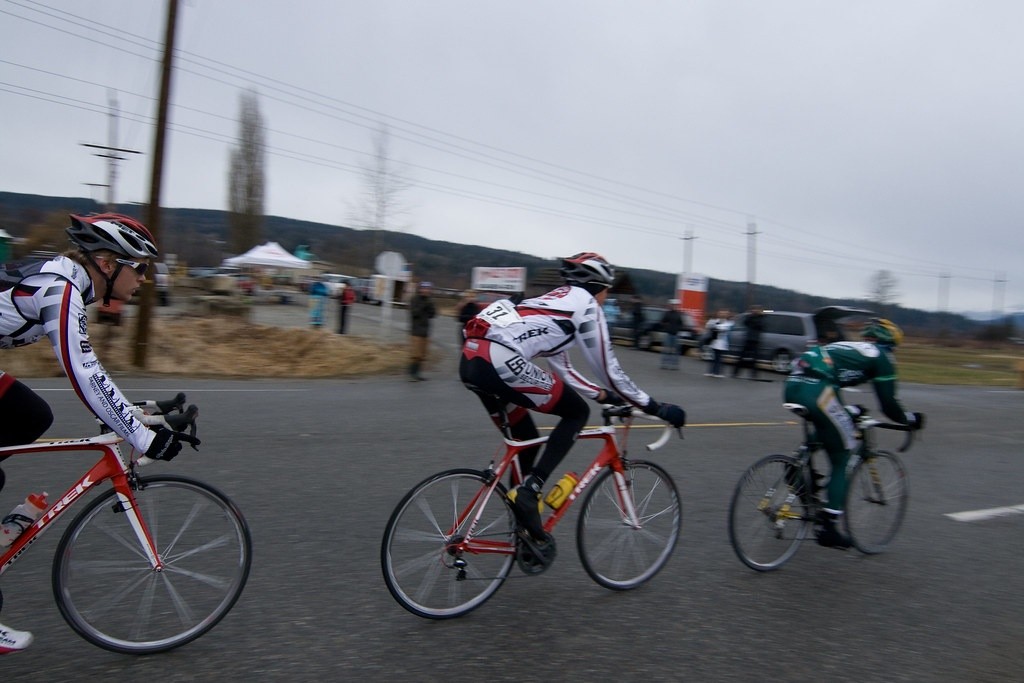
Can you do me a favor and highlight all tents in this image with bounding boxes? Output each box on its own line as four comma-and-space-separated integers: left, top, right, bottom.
222, 241, 313, 300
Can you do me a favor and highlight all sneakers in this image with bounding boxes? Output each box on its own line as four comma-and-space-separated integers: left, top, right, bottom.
0, 624, 33, 654
505, 484, 545, 541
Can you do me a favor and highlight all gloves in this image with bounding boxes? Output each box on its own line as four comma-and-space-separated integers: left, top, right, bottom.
643, 397, 685, 428
906, 412, 921, 430
597, 388, 625, 406
146, 433, 182, 461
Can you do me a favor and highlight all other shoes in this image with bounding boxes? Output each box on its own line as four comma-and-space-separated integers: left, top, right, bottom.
818, 529, 853, 548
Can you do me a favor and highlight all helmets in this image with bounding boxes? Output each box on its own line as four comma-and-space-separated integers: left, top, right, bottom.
559, 252, 614, 283
65, 212, 158, 258
862, 317, 903, 345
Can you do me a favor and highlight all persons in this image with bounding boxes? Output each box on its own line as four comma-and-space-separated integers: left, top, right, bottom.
731, 307, 770, 378
629, 294, 645, 349
0, 212, 183, 655
655, 305, 686, 371
406, 281, 436, 381
332, 279, 355, 334
703, 309, 735, 377
783, 318, 924, 549
459, 253, 687, 560
309, 278, 330, 327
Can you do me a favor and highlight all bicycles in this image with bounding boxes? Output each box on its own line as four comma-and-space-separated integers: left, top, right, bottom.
0, 391, 254, 658
378, 380, 685, 622
726, 402, 922, 575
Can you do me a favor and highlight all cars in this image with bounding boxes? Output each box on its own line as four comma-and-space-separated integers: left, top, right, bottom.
603, 307, 704, 358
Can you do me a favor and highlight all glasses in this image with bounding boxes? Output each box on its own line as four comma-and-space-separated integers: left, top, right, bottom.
95, 256, 149, 275
587, 282, 613, 289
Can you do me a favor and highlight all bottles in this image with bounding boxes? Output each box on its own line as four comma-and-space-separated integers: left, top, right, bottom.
0, 492, 49, 548
523, 467, 544, 514
543, 472, 579, 509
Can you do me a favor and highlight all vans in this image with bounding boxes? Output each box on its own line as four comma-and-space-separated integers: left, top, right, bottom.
700, 305, 877, 374
